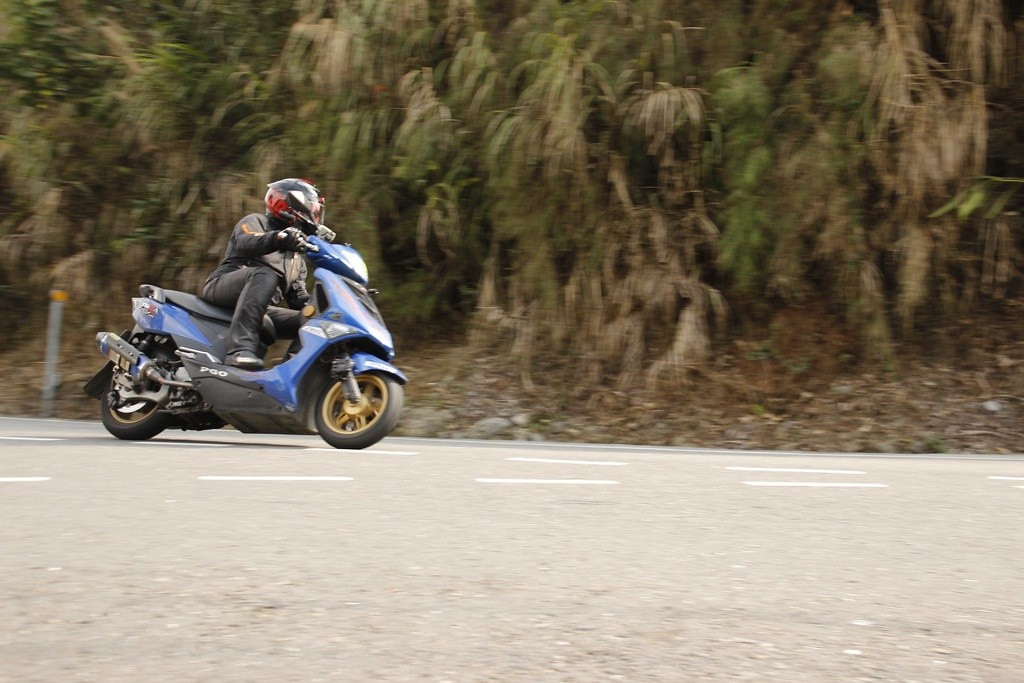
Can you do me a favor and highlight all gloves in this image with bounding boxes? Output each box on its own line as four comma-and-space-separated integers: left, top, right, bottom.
273, 226, 307, 253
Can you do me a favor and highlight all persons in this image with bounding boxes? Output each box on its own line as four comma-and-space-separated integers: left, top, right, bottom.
203, 174, 326, 369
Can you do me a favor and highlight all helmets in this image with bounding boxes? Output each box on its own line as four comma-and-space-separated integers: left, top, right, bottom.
264, 177, 324, 229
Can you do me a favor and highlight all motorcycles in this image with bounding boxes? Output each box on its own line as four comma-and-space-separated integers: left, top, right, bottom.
82, 226, 409, 450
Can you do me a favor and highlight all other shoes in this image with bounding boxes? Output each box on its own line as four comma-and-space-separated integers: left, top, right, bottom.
223, 351, 264, 370
